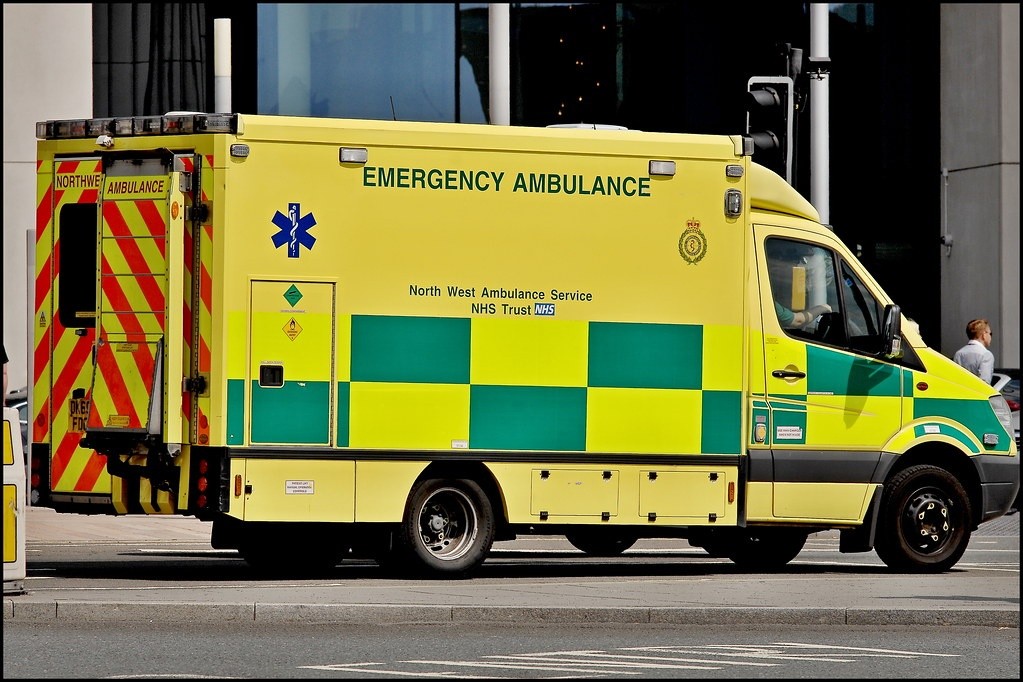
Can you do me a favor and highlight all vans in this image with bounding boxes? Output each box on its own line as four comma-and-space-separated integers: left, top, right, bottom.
20, 109, 1019, 577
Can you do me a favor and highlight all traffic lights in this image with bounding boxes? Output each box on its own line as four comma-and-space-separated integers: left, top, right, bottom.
742, 76, 792, 190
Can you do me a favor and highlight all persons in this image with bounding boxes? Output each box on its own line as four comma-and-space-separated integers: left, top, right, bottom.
772, 301, 832, 330
954, 317, 997, 388
